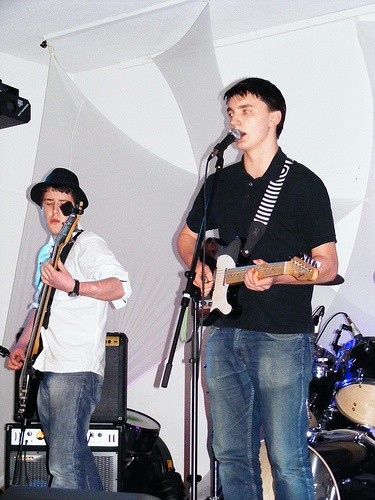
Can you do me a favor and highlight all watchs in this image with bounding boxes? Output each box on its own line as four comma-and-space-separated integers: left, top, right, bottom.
68, 279, 79, 297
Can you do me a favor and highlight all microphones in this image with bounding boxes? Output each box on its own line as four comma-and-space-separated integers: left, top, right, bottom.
312, 307, 326, 336
207, 128, 242, 162
345, 315, 364, 342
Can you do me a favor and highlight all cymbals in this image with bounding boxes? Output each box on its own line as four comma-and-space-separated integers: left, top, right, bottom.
313, 274, 346, 286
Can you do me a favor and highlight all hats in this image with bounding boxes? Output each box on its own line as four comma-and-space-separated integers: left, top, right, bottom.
30, 168, 88, 209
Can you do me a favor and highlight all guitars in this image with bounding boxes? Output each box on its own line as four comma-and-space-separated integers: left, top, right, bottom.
12, 198, 89, 427
190, 233, 321, 326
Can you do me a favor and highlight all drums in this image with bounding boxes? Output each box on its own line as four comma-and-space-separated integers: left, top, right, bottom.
305, 341, 356, 424
305, 427, 375, 500
329, 334, 375, 428
118, 407, 164, 456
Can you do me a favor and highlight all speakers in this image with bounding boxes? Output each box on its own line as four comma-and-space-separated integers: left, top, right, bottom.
4, 423, 127, 493
28, 331, 129, 426
0, 484, 161, 500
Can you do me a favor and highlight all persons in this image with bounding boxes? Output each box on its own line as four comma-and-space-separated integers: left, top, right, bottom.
176, 77, 338, 500
7, 167, 132, 491
